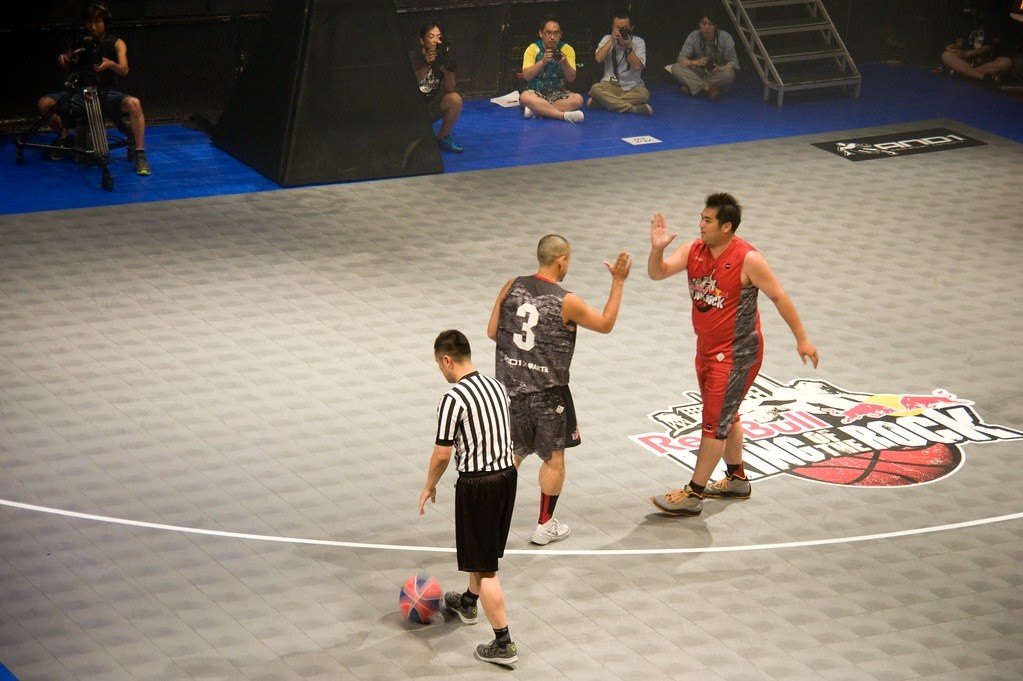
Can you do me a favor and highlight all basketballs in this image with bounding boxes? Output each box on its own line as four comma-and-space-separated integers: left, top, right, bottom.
399, 574, 443, 623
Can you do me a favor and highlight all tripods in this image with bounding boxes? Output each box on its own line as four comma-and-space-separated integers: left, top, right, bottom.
17, 72, 136, 191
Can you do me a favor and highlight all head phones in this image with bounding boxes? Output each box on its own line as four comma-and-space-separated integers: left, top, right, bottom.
78, 4, 115, 33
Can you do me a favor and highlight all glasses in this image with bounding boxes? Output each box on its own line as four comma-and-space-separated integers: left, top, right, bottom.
543, 31, 561, 36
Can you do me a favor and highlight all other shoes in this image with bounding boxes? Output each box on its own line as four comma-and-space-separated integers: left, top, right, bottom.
634, 104, 652, 116
587, 97, 602, 108
944, 67, 959, 80
680, 85, 691, 96
984, 73, 1000, 81
708, 86, 720, 100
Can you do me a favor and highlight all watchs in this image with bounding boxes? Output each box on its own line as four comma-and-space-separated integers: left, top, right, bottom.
625, 47, 633, 53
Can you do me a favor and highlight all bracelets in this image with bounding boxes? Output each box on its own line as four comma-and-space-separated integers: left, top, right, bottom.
688, 60, 693, 68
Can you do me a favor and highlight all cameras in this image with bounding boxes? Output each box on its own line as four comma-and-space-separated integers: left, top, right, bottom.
550, 49, 563, 61
617, 29, 632, 40
701, 58, 718, 71
431, 37, 457, 72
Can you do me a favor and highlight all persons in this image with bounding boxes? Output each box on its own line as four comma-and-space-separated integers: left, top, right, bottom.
407, 21, 464, 155
420, 329, 518, 663
487, 236, 633, 545
648, 193, 818, 517
941, 3, 1013, 86
521, 14, 585, 124
586, 9, 654, 116
39, 3, 151, 176
671, 7, 741, 100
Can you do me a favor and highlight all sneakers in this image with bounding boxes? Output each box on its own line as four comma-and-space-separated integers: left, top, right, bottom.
650, 485, 703, 516
564, 110, 584, 123
531, 518, 570, 544
524, 107, 537, 119
444, 591, 478, 624
48, 138, 70, 160
703, 470, 751, 498
477, 637, 518, 664
438, 135, 464, 153
135, 152, 152, 175
